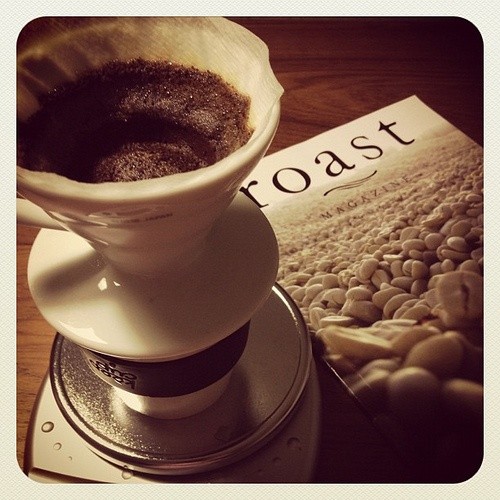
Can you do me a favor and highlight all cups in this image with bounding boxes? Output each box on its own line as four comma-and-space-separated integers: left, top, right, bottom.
16, 18, 280, 276
26, 190, 280, 420
